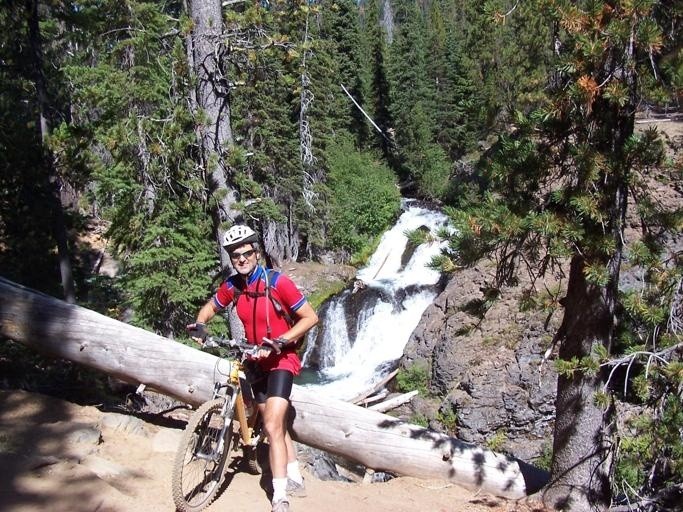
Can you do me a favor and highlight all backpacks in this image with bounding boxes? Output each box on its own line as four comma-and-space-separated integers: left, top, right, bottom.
232, 270, 306, 350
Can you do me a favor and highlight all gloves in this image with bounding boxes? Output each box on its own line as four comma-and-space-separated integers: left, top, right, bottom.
190, 322, 208, 342
257, 337, 288, 353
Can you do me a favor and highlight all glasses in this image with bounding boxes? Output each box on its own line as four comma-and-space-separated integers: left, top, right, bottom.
229, 248, 256, 259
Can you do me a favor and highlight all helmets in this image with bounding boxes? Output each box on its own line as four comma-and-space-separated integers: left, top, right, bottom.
222, 224, 258, 253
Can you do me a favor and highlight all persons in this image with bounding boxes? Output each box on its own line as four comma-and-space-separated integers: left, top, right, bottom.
183, 222, 319, 512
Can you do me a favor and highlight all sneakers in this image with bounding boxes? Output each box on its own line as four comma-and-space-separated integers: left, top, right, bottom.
271, 497, 292, 512
286, 476, 308, 497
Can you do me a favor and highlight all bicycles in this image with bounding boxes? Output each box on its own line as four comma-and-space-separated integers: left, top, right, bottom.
170, 321, 273, 512
